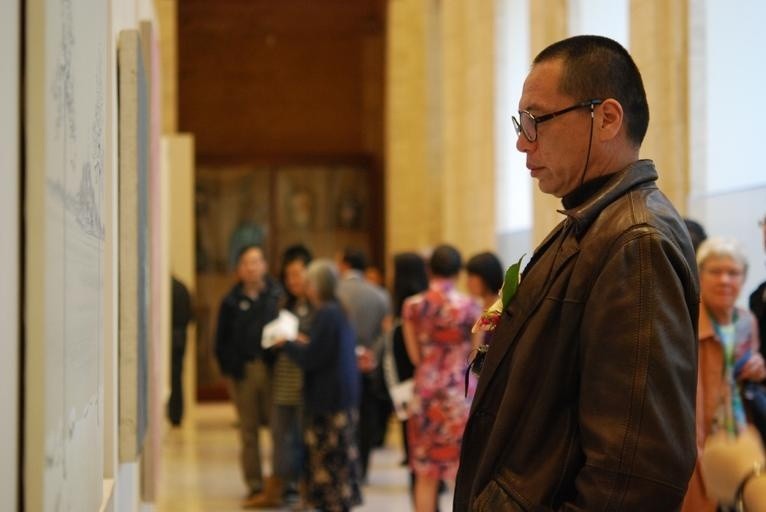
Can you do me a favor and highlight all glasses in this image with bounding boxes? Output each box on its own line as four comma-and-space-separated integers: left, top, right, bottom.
510, 97, 604, 140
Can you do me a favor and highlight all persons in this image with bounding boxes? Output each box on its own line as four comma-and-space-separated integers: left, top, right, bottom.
171, 275, 193, 426
685, 216, 706, 249
451, 35, 700, 511
679, 233, 765, 511
745, 218, 765, 438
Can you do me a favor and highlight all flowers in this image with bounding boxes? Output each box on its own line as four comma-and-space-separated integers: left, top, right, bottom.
464, 254, 526, 396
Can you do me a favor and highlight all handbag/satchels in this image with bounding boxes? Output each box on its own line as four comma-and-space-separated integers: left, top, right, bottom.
389, 378, 418, 421
